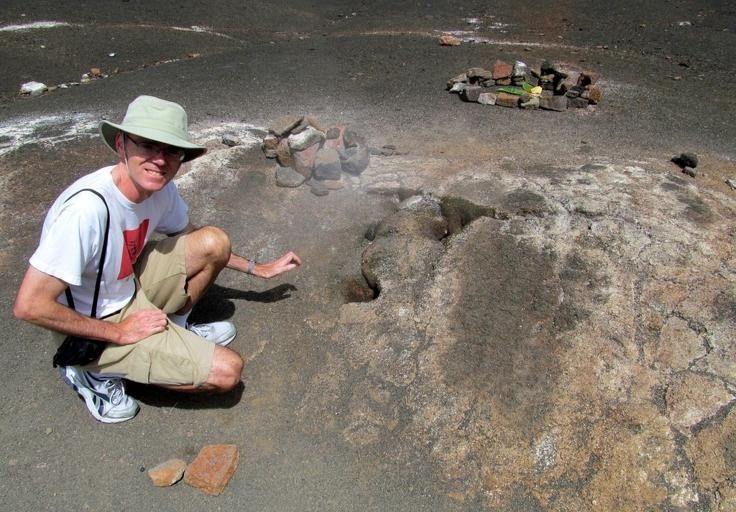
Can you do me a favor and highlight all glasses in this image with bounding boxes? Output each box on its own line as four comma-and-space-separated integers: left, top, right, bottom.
127, 135, 182, 158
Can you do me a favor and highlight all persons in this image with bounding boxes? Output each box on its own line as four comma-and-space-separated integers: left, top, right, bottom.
11, 95, 301, 423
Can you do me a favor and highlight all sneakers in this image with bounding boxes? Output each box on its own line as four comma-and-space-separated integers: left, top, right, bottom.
58, 366, 140, 423
183, 320, 237, 348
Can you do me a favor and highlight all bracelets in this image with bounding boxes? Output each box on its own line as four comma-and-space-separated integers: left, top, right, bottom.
246, 258, 257, 275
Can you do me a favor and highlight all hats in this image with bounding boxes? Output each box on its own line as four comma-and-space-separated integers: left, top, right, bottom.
99, 95, 206, 165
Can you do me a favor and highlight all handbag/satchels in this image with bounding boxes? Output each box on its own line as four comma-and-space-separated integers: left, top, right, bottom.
52, 333, 110, 370
